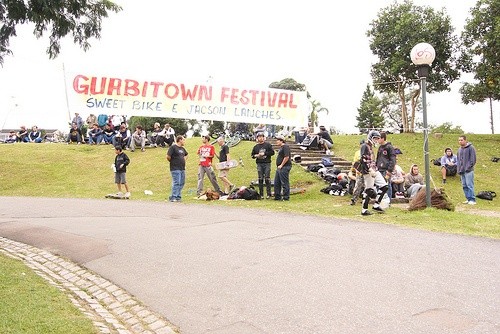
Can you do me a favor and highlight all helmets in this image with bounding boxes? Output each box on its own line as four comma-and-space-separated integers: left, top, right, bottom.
368, 129, 381, 145
256, 132, 264, 138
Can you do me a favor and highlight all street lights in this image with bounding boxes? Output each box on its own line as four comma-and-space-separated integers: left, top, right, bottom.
410, 42, 436, 206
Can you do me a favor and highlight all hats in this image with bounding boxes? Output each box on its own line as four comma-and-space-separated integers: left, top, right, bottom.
275, 135, 286, 143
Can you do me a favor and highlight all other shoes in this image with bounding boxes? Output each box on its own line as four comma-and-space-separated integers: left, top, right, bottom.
361, 209, 370, 215
443, 179, 446, 184
141, 148, 145, 151
372, 206, 383, 212
131, 148, 134, 152
468, 201, 476, 204
260, 195, 264, 198
170, 197, 177, 201
267, 195, 270, 199
463, 200, 469, 204
125, 192, 131, 197
118, 192, 122, 197
78, 141, 80, 144
125, 147, 129, 150
176, 196, 181, 202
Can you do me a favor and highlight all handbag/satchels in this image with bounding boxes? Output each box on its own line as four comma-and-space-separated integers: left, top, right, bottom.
476, 191, 497, 200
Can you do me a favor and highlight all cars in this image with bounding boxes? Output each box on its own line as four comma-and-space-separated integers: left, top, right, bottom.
0, 131, 66, 143
360, 123, 403, 135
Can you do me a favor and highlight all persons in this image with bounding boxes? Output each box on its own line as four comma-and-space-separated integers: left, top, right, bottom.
167, 135, 189, 202
251, 132, 275, 199
348, 129, 424, 215
441, 148, 457, 186
214, 137, 235, 194
273, 135, 292, 201
5, 126, 43, 143
65, 113, 177, 152
197, 136, 224, 196
112, 145, 131, 197
253, 124, 333, 153
457, 135, 477, 204
